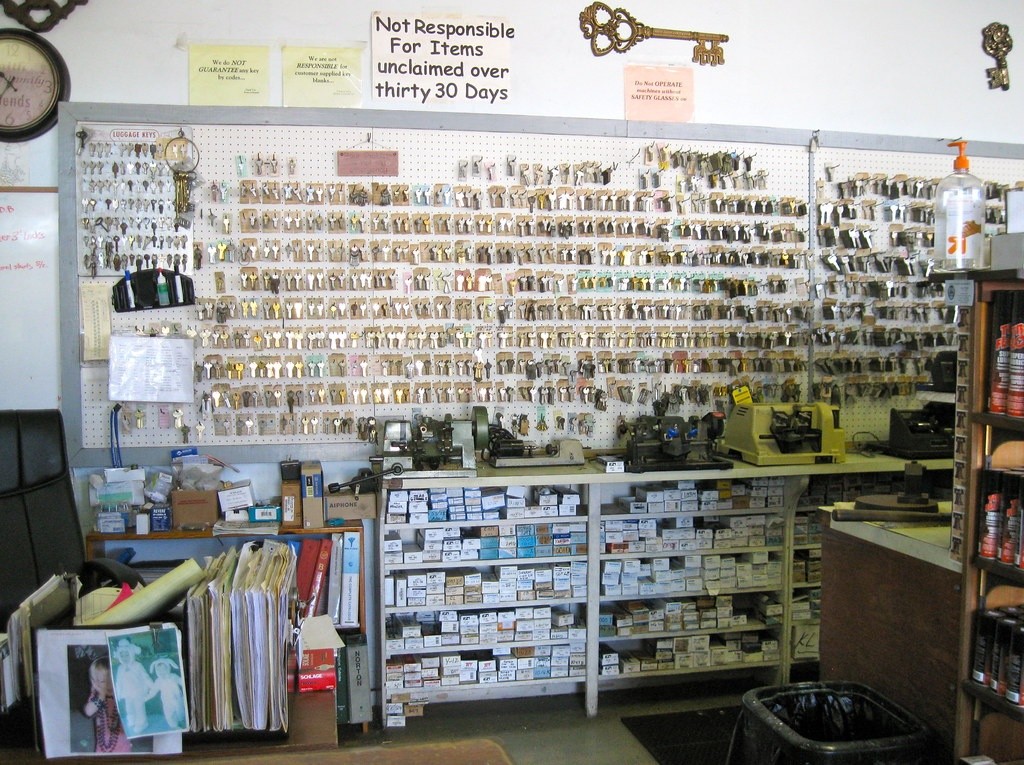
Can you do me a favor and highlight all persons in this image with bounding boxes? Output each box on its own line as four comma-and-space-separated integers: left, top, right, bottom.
84, 655, 132, 752
144, 658, 186, 729
112, 639, 153, 733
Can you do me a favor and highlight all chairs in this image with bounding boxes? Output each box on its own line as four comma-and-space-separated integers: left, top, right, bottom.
0, 408, 185, 632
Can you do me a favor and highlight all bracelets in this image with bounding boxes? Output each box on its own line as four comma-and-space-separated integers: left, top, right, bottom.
91, 695, 104, 708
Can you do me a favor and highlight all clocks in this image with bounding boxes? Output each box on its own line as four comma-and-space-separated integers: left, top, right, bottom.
0, 27, 71, 143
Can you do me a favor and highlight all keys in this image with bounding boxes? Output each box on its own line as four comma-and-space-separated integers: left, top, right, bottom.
981, 21, 1013, 90
75, 128, 1016, 444
578, 1, 729, 66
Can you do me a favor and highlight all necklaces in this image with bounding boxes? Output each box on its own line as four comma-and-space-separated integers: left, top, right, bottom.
97, 695, 120, 752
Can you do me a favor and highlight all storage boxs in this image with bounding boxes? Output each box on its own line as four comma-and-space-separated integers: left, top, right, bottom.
322, 486, 378, 522
224, 507, 250, 523
249, 498, 281, 524
218, 479, 258, 512
171, 483, 222, 531
301, 460, 326, 528
281, 478, 302, 529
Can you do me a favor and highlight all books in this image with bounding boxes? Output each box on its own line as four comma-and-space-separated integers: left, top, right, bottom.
288, 531, 360, 626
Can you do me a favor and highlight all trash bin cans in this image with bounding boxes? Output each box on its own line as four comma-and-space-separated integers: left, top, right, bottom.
724, 677, 933, 765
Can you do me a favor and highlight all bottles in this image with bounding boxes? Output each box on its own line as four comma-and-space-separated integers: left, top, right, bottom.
968, 287, 1024, 709
156, 268, 170, 307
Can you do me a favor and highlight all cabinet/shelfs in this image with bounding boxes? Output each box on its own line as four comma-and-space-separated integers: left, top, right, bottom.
929, 268, 1024, 765
373, 459, 956, 728
86, 519, 370, 744
